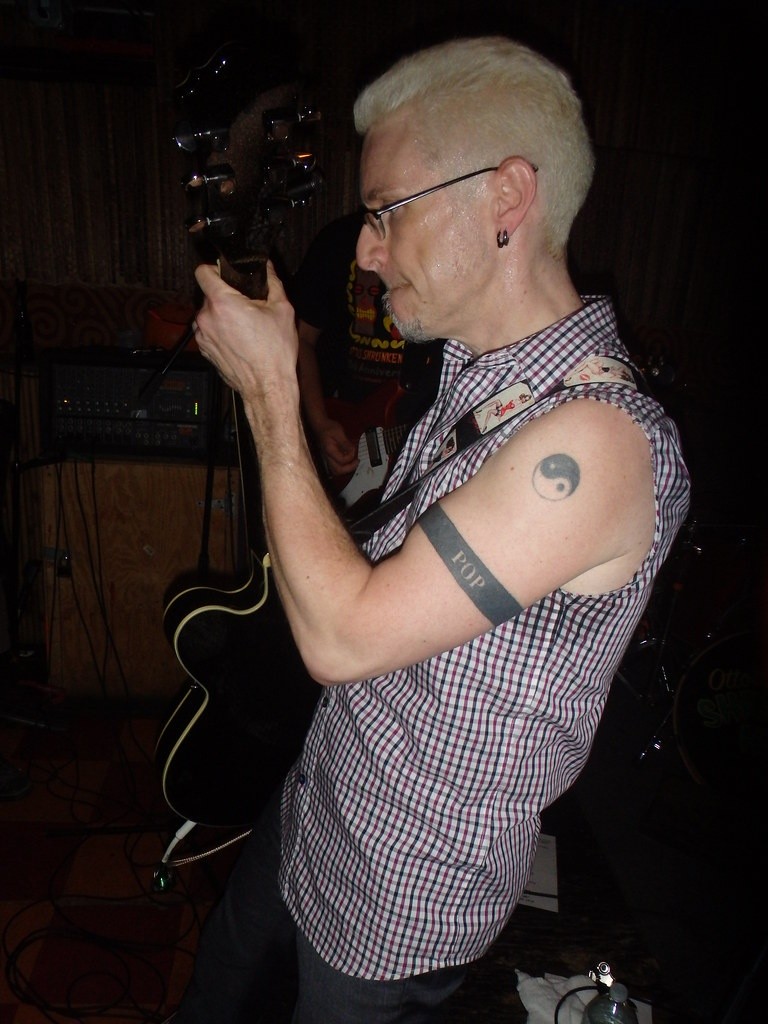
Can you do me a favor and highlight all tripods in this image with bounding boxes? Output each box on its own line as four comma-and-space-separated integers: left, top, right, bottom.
614, 513, 703, 710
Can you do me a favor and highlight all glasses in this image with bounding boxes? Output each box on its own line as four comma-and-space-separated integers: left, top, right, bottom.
359, 156, 539, 241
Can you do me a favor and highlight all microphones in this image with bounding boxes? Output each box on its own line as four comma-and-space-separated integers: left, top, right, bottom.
16, 280, 36, 366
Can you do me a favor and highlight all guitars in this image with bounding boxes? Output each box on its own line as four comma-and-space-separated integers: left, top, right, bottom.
158, 47, 327, 872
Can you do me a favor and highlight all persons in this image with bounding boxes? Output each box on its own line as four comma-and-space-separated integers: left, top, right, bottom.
191, 35, 693, 1024
279, 206, 448, 480
